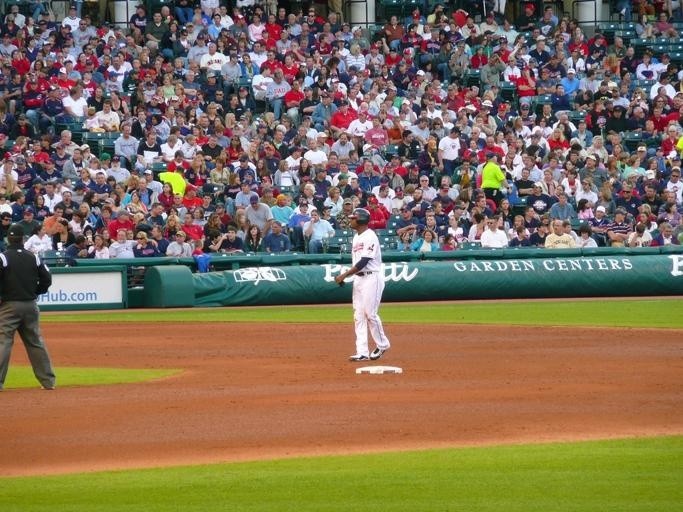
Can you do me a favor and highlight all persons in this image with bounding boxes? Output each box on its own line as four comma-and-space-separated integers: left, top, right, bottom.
1, 222, 56, 392
335, 210, 391, 362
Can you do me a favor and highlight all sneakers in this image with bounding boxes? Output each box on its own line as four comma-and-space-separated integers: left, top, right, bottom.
371, 346, 386, 360
349, 355, 370, 361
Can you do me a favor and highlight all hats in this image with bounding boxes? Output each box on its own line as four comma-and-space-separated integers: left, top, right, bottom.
482, 98, 647, 215
7, 224, 25, 236
15, 4, 154, 92
340, 22, 424, 105
16, 145, 187, 238
238, 156, 411, 213
182, 23, 242, 78
525, 4, 612, 77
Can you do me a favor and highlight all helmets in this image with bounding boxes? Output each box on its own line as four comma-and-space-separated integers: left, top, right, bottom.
348, 208, 371, 225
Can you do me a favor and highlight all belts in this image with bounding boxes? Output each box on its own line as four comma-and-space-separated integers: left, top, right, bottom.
358, 272, 373, 277
